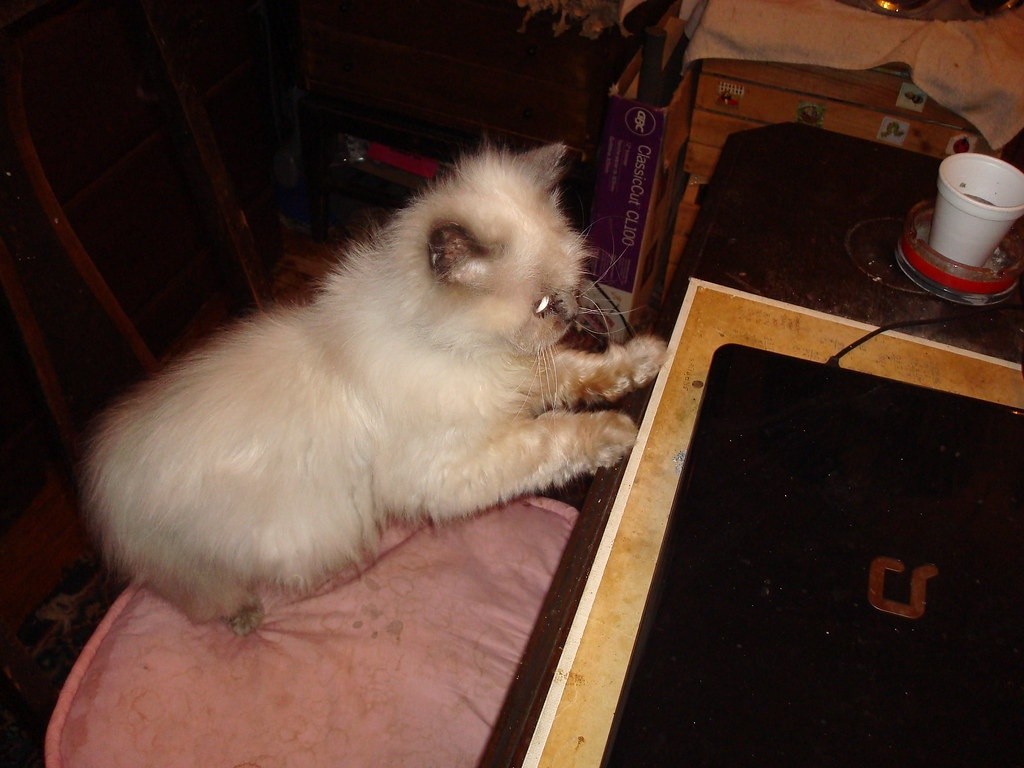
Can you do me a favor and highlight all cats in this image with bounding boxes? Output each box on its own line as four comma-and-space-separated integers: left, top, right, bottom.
78, 141, 668, 624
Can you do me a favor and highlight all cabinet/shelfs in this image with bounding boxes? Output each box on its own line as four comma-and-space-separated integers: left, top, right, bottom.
663, 0, 1024, 313
300, 0, 673, 243
0, 0, 284, 542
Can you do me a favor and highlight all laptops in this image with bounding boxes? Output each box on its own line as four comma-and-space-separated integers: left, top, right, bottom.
600, 343, 1024, 768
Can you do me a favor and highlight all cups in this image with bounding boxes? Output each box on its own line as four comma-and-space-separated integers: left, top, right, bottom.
930, 153, 1024, 267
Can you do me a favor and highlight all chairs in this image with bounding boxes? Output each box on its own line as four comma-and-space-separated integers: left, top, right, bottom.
1, 0, 579, 768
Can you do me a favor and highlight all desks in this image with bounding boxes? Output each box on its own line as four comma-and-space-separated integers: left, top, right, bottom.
477, 123, 1023, 768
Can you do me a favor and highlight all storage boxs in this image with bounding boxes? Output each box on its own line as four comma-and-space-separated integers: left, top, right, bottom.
584, 1, 700, 346
